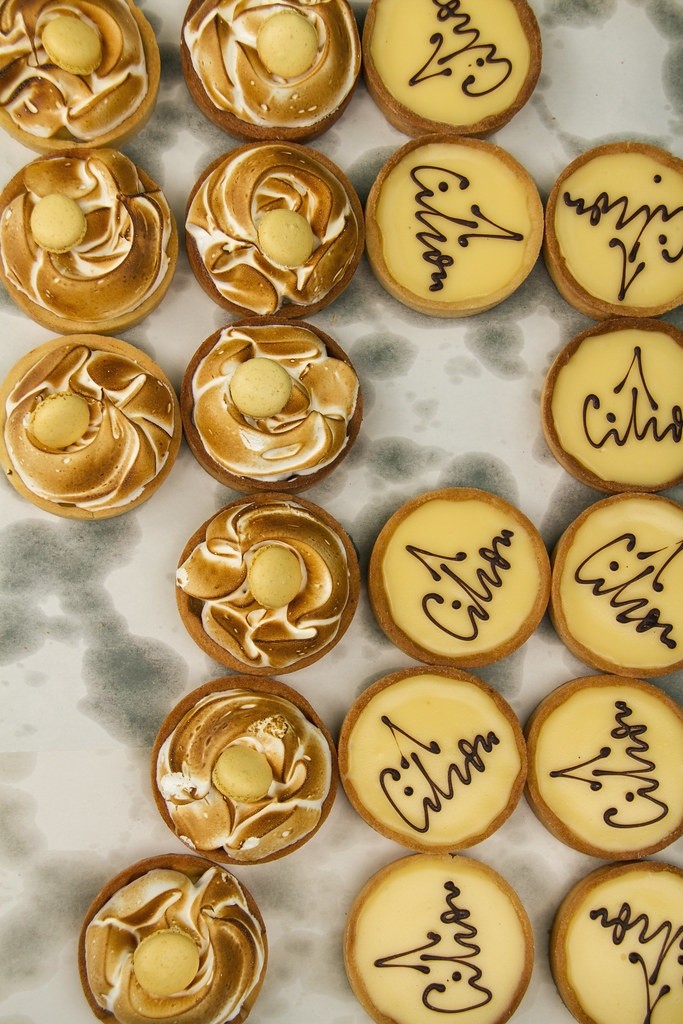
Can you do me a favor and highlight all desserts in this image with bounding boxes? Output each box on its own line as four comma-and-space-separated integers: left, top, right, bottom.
338, 0, 683, 1024
0, 1, 363, 1024
